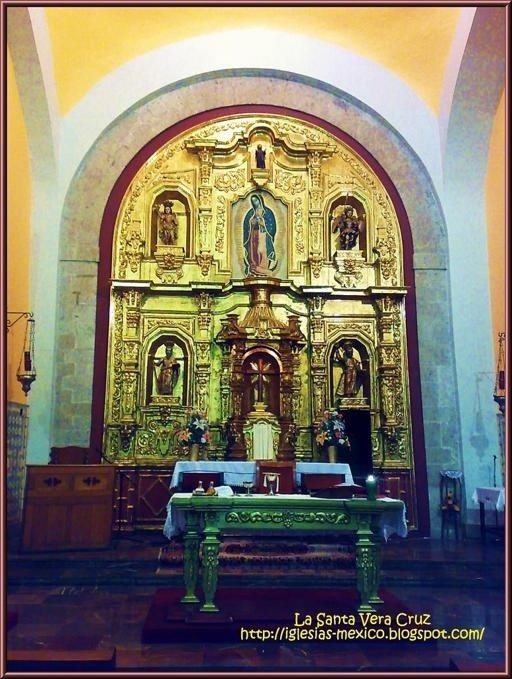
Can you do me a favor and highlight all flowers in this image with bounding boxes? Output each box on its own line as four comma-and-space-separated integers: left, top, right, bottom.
439, 490, 462, 519
310, 405, 354, 453
186, 413, 210, 445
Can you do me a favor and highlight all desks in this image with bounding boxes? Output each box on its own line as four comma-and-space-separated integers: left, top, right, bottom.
165, 488, 414, 616
475, 484, 505, 562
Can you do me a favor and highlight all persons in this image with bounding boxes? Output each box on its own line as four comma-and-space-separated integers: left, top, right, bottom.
242, 192, 279, 280
254, 144, 267, 169
333, 207, 365, 248
335, 343, 361, 396
154, 346, 180, 395
157, 204, 178, 244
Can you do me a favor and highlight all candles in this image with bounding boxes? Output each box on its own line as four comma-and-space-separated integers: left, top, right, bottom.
499, 370, 505, 390
364, 473, 379, 502
23, 350, 32, 372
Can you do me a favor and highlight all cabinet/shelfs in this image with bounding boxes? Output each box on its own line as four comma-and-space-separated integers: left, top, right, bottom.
18, 462, 121, 553
439, 470, 468, 545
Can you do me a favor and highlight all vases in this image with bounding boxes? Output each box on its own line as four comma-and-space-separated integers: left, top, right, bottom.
189, 443, 200, 461
327, 445, 338, 464
